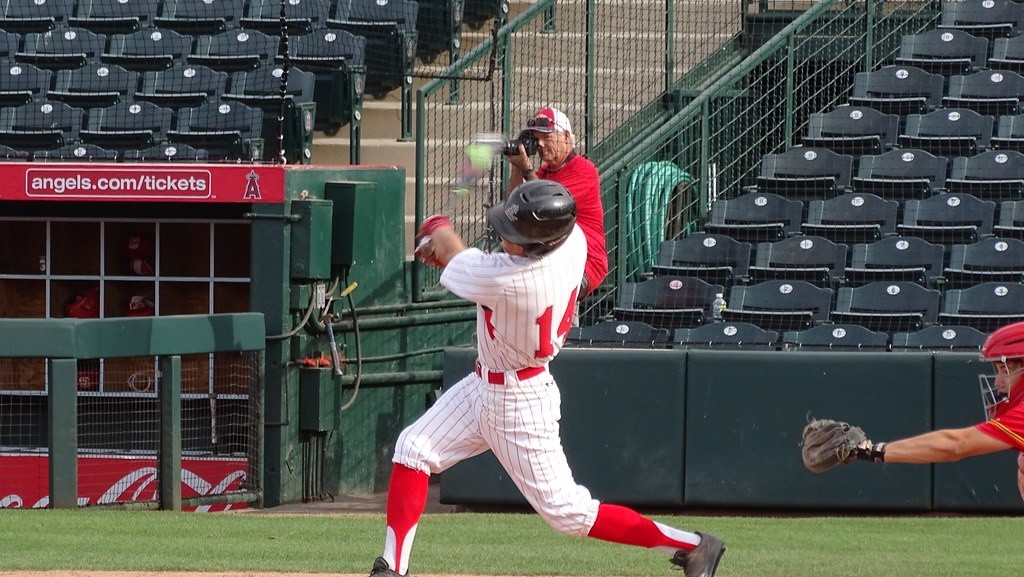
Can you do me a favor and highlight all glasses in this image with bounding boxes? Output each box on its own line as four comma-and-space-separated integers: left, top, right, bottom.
527, 118, 566, 131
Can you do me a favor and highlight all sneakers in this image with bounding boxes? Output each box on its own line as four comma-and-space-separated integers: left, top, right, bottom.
669, 531, 726, 577
369, 557, 410, 577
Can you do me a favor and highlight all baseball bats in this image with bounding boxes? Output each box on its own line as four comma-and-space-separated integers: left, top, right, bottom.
417, 132, 506, 256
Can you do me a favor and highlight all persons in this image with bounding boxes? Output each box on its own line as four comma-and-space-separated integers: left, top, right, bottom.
801, 322, 1024, 502
507, 107, 609, 327
370, 179, 726, 577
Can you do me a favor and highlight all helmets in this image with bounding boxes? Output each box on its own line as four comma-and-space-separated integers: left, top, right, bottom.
978, 322, 1024, 422
487, 180, 577, 251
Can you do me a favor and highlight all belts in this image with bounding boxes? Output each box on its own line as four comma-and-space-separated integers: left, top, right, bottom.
475, 360, 546, 386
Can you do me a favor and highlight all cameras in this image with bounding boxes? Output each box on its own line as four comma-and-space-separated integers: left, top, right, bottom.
501, 134, 538, 157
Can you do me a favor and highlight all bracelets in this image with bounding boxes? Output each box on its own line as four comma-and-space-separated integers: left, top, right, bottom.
523, 168, 534, 180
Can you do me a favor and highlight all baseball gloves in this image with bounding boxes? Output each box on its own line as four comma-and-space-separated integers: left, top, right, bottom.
799, 418, 871, 474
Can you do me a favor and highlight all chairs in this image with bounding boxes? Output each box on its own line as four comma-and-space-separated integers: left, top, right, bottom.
0, 0, 1023, 354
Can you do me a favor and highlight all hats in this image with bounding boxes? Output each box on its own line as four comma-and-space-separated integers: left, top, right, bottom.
524, 108, 571, 134
